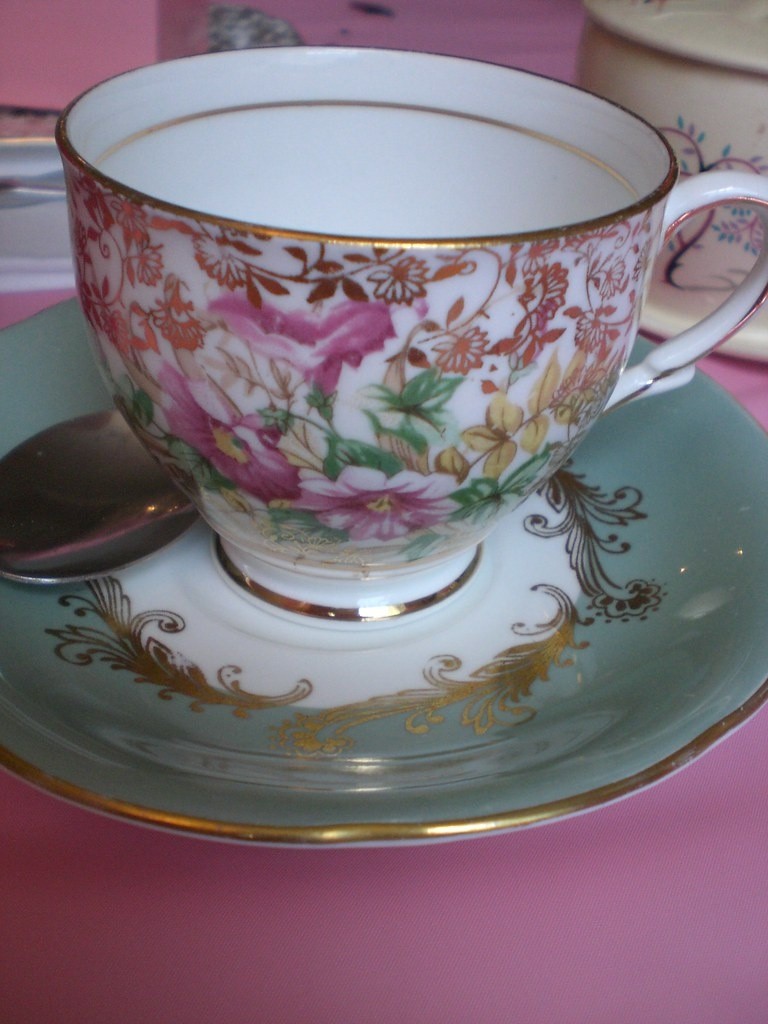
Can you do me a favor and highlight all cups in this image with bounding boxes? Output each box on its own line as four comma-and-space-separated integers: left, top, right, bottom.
55, 48, 768, 623
582, 0, 768, 363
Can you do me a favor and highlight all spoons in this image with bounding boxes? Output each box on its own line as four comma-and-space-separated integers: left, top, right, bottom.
0, 408, 200, 586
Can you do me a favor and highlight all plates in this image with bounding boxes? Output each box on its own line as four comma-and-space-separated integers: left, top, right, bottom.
0, 294, 768, 850
